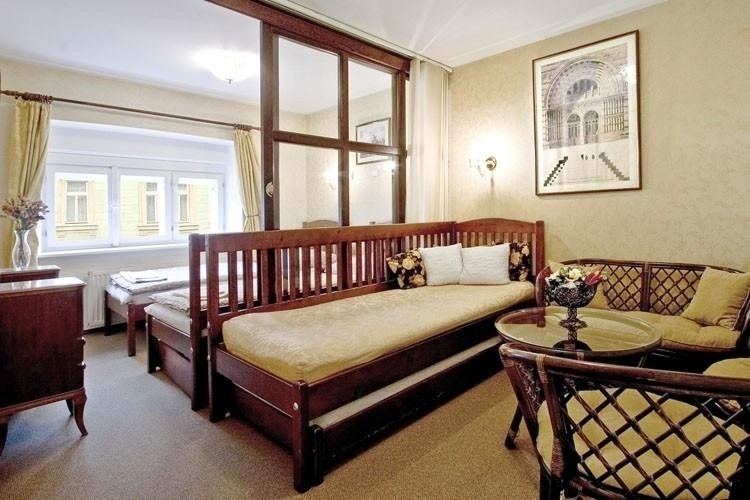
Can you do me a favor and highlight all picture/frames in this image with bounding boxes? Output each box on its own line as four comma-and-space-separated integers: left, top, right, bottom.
527, 28, 644, 197
354, 117, 393, 168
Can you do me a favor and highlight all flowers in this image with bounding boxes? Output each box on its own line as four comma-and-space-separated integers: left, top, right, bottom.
1, 192, 52, 266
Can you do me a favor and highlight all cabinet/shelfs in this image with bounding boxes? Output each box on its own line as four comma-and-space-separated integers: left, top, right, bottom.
0, 264, 90, 455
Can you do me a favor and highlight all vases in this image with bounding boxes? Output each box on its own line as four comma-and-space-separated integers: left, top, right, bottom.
9, 230, 33, 271
